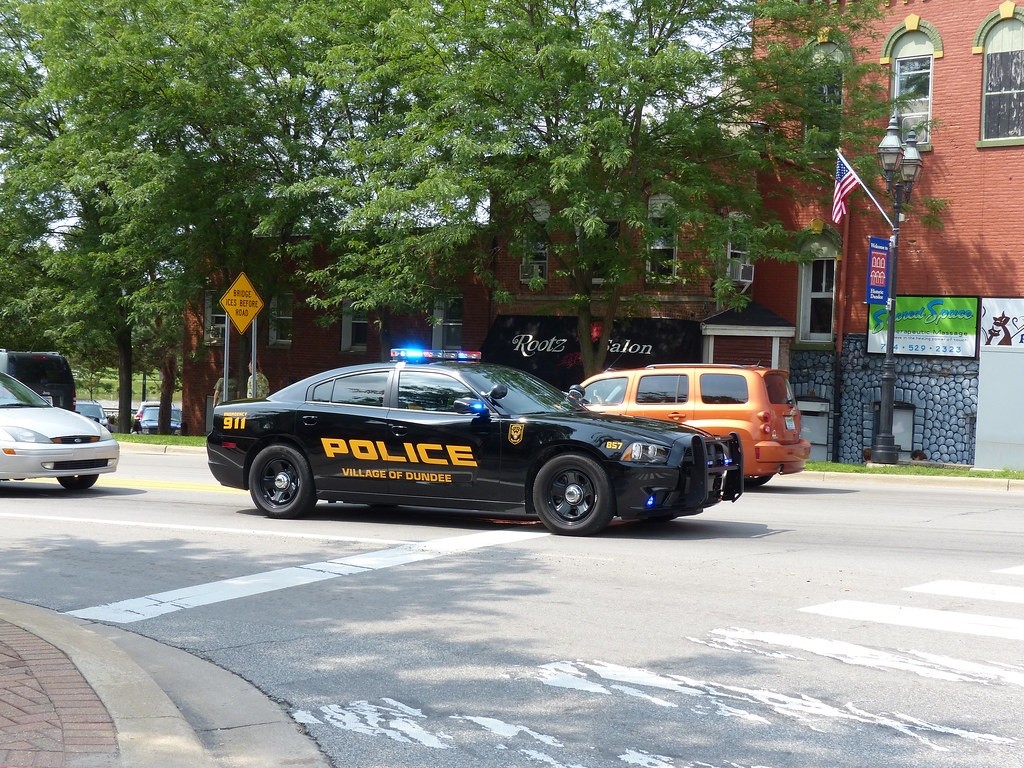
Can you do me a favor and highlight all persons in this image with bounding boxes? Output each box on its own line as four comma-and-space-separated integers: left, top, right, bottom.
246, 359, 270, 400
107, 415, 117, 432
213, 367, 240, 409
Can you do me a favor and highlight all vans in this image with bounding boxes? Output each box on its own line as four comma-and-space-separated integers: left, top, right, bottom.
0, 347, 77, 413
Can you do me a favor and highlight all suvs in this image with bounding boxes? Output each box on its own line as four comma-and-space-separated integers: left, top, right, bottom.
560, 361, 811, 490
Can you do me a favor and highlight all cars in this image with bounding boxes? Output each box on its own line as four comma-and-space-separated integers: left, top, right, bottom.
132, 399, 183, 436
204, 347, 746, 538
76, 401, 110, 432
0, 371, 120, 490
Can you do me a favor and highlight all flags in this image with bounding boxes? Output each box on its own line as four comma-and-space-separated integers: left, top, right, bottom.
832, 156, 859, 224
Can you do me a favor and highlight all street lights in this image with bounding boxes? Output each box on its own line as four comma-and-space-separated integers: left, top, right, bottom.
874, 107, 935, 464
